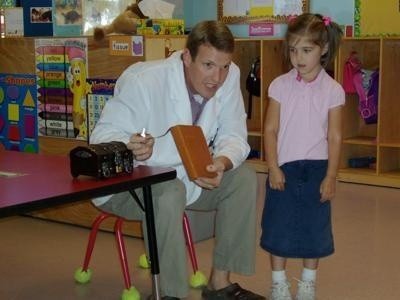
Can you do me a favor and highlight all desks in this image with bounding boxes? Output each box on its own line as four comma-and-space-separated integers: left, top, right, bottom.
0, 150, 176, 299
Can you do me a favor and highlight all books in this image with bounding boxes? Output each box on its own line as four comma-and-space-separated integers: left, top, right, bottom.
169, 125, 218, 182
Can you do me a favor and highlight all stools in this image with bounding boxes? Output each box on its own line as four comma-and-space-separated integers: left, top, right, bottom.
73, 211, 209, 299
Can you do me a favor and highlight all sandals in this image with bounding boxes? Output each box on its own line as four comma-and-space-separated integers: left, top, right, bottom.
201, 282, 267, 300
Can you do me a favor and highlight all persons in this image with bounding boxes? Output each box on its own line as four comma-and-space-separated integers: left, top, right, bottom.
256, 11, 347, 300
32, 8, 51, 22
83, 19, 269, 300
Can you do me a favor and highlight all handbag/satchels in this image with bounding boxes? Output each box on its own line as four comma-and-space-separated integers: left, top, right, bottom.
244, 59, 260, 98
342, 51, 362, 96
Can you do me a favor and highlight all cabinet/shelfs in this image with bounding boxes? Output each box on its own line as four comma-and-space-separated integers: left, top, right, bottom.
231, 37, 400, 190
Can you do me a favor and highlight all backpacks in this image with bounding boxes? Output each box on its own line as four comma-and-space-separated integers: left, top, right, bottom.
353, 67, 379, 124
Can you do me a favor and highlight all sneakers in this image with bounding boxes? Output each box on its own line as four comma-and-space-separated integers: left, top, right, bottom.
147, 294, 181, 300
270, 278, 292, 300
295, 281, 316, 299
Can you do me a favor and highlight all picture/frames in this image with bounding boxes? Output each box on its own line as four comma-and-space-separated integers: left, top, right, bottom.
217, 0, 309, 23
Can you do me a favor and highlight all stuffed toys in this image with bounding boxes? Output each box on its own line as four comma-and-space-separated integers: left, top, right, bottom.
94, 0, 149, 42
